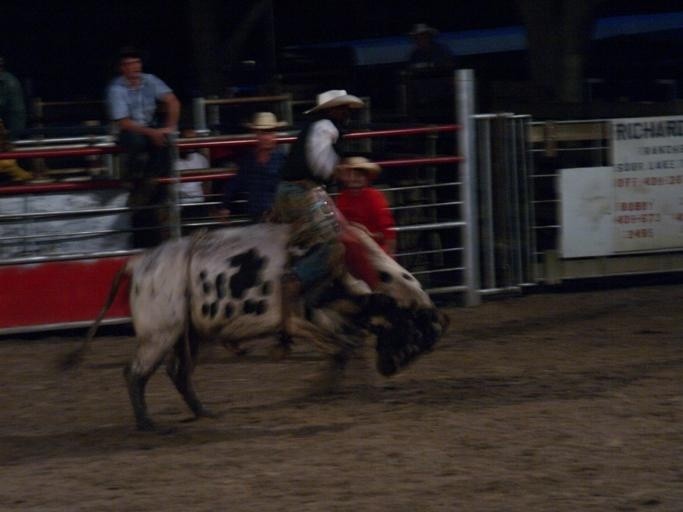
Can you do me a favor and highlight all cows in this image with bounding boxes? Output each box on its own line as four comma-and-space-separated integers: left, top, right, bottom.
64, 216, 449, 432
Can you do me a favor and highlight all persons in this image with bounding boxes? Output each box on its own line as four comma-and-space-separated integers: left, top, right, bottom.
214, 111, 296, 361
321, 157, 399, 370
271, 89, 365, 316
1, 21, 682, 246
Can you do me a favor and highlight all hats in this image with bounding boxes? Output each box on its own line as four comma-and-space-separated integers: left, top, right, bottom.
303, 90, 363, 115
242, 113, 289, 130
336, 157, 380, 181
